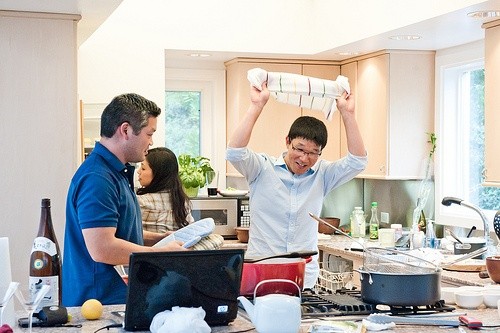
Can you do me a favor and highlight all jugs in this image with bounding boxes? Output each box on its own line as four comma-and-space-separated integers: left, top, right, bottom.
237, 278, 302, 333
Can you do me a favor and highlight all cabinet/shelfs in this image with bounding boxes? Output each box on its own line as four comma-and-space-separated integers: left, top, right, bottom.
225, 48, 435, 180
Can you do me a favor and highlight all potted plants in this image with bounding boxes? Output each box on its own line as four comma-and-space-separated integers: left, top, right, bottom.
177, 154, 214, 198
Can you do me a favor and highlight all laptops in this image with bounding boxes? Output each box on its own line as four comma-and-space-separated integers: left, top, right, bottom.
111, 248, 245, 331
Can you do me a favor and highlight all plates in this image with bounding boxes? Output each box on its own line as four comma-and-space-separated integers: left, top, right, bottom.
219, 191, 248, 196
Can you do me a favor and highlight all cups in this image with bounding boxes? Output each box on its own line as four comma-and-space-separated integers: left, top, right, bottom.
378, 228, 395, 248
205, 171, 219, 197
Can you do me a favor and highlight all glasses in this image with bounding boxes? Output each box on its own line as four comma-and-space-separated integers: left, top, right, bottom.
290, 138, 322, 158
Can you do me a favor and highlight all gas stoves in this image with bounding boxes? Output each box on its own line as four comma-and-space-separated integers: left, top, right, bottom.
236, 286, 456, 324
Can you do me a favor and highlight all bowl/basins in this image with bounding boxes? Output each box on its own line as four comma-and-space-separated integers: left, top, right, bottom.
486, 256, 500, 283
439, 283, 500, 310
233, 227, 249, 243
318, 217, 340, 235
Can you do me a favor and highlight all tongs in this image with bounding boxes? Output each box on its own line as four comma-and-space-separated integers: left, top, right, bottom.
366, 313, 459, 328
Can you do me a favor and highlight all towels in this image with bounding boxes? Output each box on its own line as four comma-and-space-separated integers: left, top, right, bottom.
246, 68, 351, 122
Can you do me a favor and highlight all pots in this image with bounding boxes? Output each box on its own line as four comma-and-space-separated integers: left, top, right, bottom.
351, 264, 443, 307
454, 242, 486, 260
240, 255, 313, 296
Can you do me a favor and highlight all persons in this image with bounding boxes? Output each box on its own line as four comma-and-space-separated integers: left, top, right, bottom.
225, 82, 369, 293
61, 93, 194, 308
136, 148, 195, 234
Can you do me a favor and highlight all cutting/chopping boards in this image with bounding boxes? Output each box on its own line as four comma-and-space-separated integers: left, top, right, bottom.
441, 258, 487, 271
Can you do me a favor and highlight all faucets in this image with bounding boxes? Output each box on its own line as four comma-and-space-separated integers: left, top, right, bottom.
441, 195, 490, 247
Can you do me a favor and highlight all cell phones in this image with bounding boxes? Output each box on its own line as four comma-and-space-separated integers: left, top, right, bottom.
19, 317, 41, 327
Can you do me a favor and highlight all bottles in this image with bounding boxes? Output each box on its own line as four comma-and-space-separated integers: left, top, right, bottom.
416, 198, 426, 235
390, 223, 403, 242
350, 207, 366, 238
28, 198, 62, 317
369, 202, 379, 240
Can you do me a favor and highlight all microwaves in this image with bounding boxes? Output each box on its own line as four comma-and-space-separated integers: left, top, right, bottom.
186, 195, 250, 240
